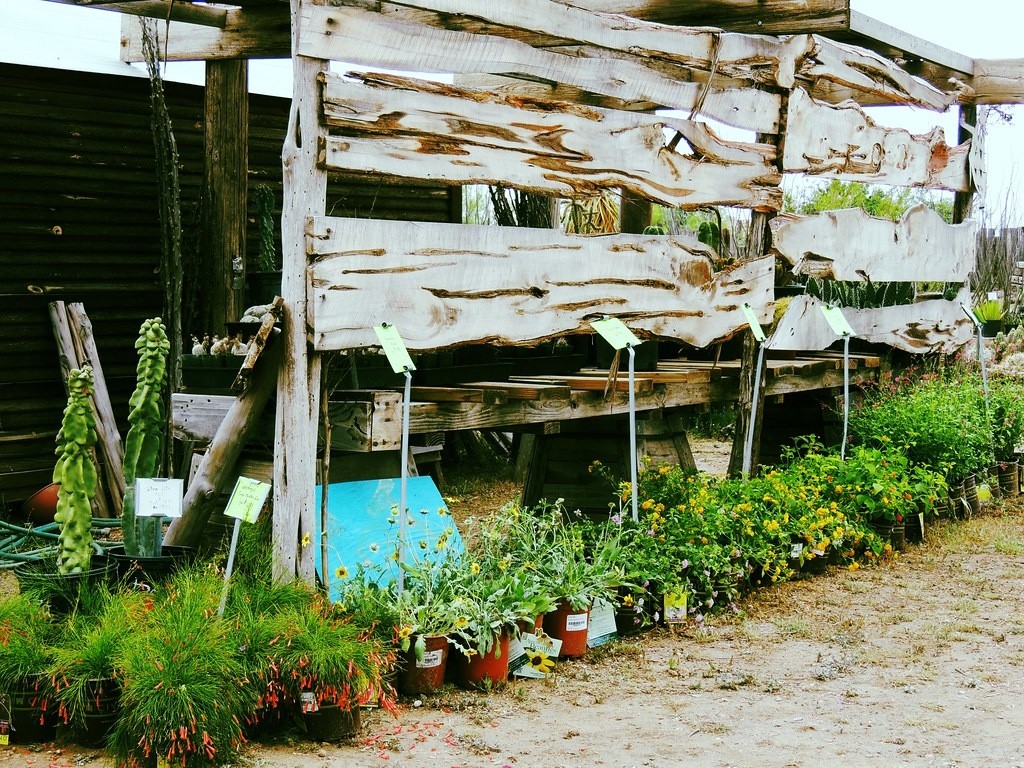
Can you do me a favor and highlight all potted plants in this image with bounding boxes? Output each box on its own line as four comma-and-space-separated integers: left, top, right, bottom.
97, 607, 258, 768
31, 558, 149, 750
215, 610, 295, 743
276, 606, 409, 745
0, 587, 65, 745
104, 316, 195, 590
14, 364, 120, 625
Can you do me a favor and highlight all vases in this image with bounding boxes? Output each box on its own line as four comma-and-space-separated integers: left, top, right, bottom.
998, 457, 1019, 497
615, 607, 641, 637
873, 523, 893, 542
20, 483, 61, 534
963, 474, 982, 514
891, 523, 905, 549
950, 485, 964, 518
988, 464, 1000, 499
515, 614, 544, 634
543, 598, 591, 657
455, 629, 510, 691
712, 580, 738, 607
905, 500, 925, 542
403, 634, 451, 693
807, 545, 833, 576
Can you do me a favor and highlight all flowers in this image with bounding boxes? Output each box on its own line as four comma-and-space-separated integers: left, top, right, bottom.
299, 349, 1024, 672
